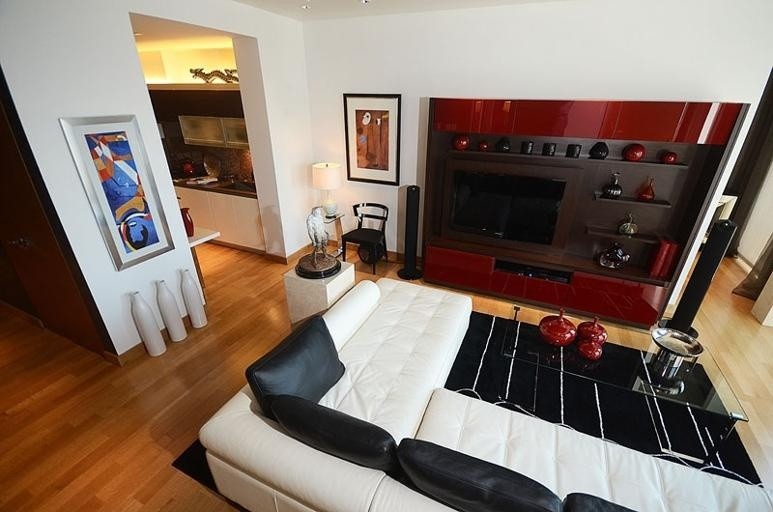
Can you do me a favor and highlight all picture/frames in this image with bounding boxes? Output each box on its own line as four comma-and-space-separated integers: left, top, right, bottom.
59, 114, 175, 273
343, 93, 402, 186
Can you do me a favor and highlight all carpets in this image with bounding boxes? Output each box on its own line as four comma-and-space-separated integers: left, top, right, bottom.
447, 313, 764, 487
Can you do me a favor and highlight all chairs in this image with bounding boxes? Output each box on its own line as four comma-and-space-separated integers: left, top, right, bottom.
341, 203, 390, 275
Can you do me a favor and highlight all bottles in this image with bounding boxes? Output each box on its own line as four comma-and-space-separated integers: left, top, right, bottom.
128, 268, 208, 358
539, 307, 608, 361
588, 141, 676, 200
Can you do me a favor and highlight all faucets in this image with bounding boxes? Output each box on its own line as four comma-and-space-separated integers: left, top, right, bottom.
229, 173, 236, 184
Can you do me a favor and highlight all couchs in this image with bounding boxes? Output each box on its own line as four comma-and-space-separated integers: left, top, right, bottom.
197, 277, 773, 512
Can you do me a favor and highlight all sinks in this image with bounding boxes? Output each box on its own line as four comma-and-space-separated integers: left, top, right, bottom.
217, 180, 257, 195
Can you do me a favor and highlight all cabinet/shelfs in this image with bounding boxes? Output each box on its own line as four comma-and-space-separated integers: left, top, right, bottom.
422, 243, 670, 330
174, 183, 265, 254
448, 149, 690, 245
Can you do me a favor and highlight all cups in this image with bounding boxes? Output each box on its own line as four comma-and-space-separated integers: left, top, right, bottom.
542, 143, 556, 155
566, 144, 582, 158
521, 141, 534, 154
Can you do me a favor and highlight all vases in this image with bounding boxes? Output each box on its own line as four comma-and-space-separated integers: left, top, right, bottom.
180, 207, 194, 237
130, 269, 208, 358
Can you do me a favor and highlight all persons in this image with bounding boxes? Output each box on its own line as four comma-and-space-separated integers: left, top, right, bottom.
306, 207, 330, 265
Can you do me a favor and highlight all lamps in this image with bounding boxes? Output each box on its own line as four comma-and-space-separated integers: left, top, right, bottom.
311, 162, 342, 218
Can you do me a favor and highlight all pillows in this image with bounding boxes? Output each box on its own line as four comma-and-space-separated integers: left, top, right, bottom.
559, 492, 637, 510
267, 396, 409, 485
396, 439, 562, 510
246, 313, 347, 421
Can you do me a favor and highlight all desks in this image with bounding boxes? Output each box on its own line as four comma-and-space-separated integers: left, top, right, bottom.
188, 228, 221, 288
